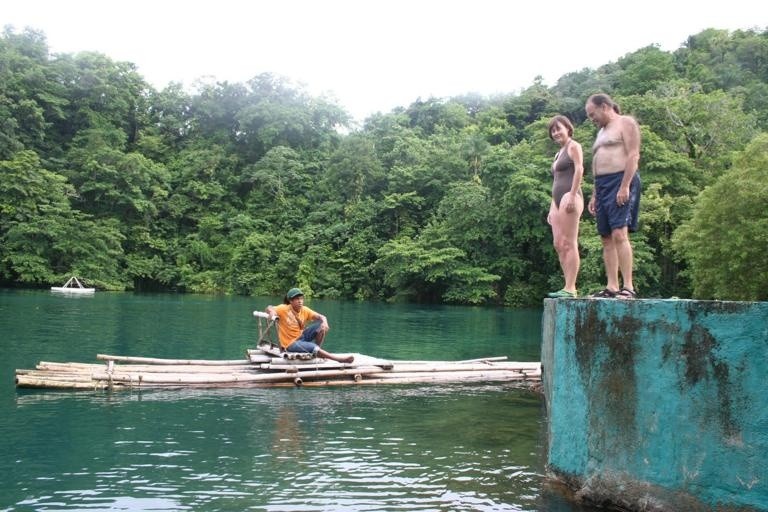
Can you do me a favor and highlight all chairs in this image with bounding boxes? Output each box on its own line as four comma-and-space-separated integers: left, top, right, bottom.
253, 310, 313, 360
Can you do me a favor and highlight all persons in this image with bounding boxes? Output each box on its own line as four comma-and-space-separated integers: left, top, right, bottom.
262, 287, 355, 364
545, 115, 584, 298
584, 93, 642, 299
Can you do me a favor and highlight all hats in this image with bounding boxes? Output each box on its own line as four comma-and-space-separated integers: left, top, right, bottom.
286, 288, 303, 300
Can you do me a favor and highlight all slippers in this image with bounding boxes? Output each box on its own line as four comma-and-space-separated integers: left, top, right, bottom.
548, 289, 574, 297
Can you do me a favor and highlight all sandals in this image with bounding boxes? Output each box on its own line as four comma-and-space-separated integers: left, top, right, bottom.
615, 287, 633, 299
591, 288, 620, 298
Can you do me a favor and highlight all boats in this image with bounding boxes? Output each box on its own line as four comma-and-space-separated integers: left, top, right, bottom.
11, 346, 545, 399
50, 276, 95, 294
